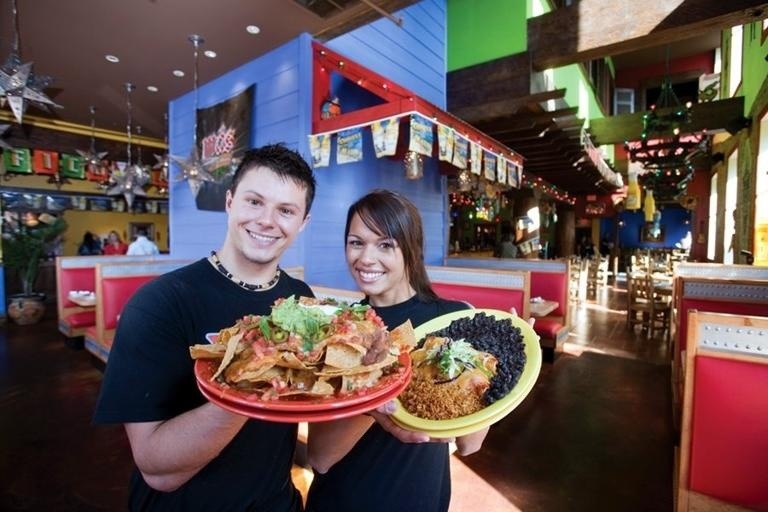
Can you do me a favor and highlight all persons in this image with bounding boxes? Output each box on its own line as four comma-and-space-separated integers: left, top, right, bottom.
494, 219, 514, 257
103, 231, 129, 254
77, 229, 100, 255
302, 186, 542, 512
125, 225, 161, 255
91, 140, 375, 512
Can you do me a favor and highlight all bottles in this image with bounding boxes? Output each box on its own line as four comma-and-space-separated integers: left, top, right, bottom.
450, 192, 495, 224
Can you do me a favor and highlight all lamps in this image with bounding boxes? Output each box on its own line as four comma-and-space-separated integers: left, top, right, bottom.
0, 0, 221, 209
456, 169, 472, 192
623, 44, 713, 200
405, 151, 425, 179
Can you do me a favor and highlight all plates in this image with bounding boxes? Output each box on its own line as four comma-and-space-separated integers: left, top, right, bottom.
192, 332, 414, 426
382, 305, 544, 441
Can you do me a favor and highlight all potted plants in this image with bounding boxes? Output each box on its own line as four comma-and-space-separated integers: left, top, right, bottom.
0, 217, 68, 326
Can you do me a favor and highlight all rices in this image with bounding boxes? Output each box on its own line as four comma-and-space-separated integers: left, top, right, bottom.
396, 350, 483, 419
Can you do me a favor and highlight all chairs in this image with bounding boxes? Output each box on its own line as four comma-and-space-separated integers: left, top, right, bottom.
549, 246, 699, 339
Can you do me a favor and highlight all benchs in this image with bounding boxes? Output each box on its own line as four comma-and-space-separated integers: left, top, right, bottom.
425, 257, 576, 364
668, 260, 768, 512
53, 255, 365, 366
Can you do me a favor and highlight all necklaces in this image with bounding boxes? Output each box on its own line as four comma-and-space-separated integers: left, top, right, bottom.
210, 250, 281, 291
